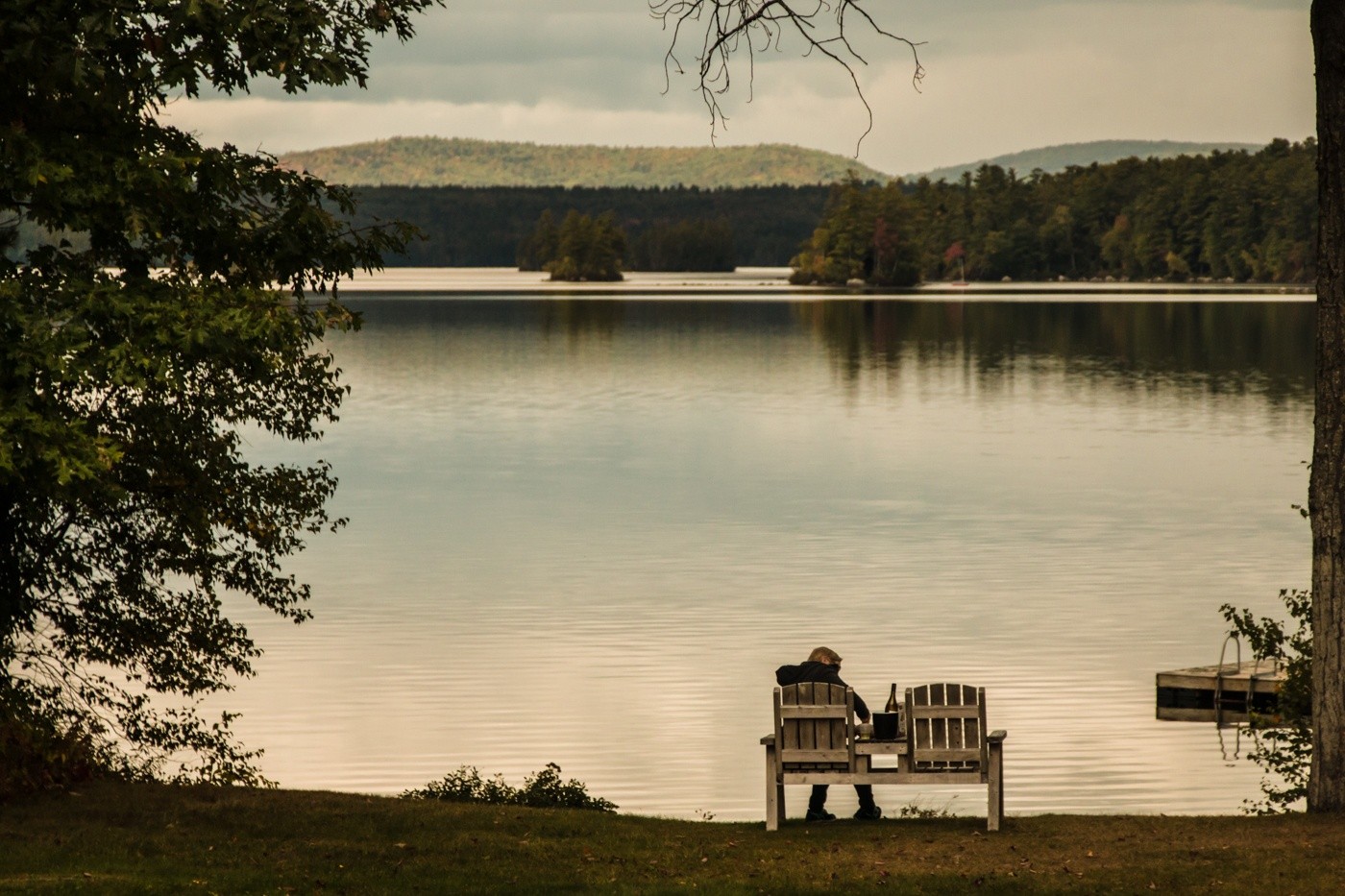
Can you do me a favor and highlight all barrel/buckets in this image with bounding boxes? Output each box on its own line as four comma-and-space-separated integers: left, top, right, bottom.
872, 712, 899, 740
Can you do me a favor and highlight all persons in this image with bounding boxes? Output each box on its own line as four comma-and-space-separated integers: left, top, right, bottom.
775, 647, 882, 819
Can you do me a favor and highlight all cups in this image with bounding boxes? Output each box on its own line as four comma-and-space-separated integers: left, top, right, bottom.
859, 724, 871, 740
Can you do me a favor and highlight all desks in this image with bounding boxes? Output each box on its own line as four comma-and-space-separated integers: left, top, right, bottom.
853, 737, 912, 754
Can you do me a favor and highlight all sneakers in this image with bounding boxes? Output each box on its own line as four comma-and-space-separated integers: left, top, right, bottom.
855, 805, 882, 820
806, 807, 835, 824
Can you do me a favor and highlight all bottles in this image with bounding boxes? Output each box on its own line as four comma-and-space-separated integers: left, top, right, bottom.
885, 683, 899, 713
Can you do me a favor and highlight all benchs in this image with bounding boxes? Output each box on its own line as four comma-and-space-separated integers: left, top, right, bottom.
760, 683, 1008, 832
